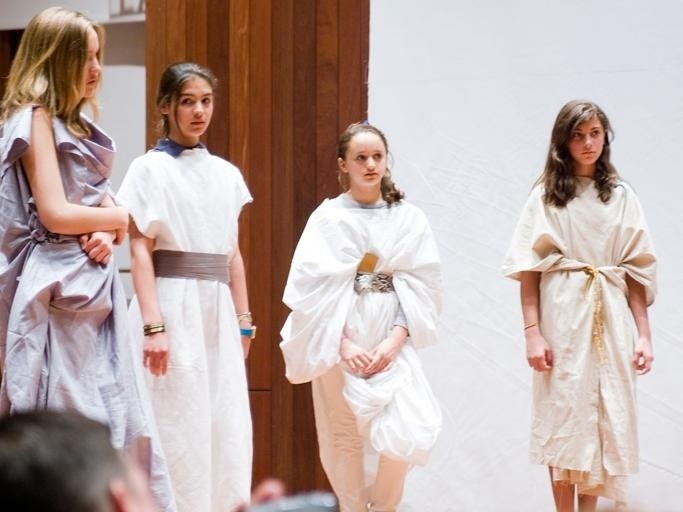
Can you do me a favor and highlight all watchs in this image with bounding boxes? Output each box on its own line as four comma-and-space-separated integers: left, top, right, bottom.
241, 326, 257, 339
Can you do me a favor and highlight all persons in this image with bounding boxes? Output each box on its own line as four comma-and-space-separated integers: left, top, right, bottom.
1, 407, 287, 511
111, 60, 257, 512
278, 122, 444, 512
502, 98, 659, 512
0, 6, 177, 512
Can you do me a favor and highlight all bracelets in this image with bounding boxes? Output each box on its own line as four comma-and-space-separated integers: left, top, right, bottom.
142, 322, 166, 336
237, 312, 254, 323
523, 320, 539, 331
